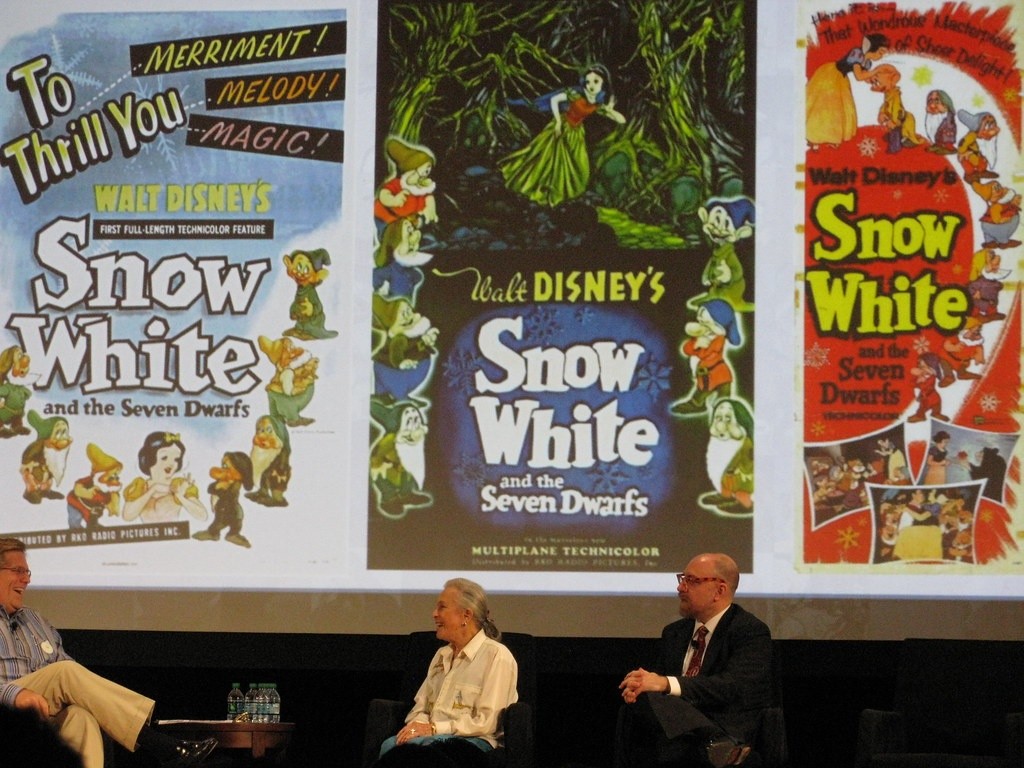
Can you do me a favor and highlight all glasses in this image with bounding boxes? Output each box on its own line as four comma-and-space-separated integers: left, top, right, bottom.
677, 572, 727, 587
0, 565, 32, 579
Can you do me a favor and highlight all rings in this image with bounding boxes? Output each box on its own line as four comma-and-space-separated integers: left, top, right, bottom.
411, 728, 416, 734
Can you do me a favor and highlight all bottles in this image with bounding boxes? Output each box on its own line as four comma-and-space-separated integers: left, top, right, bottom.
252, 684, 280, 723
227, 683, 245, 721
244, 683, 257, 720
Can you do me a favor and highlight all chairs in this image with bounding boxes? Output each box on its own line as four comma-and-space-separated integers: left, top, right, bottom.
1, 706, 114, 768
609, 640, 789, 768
855, 638, 1023, 768
363, 632, 537, 768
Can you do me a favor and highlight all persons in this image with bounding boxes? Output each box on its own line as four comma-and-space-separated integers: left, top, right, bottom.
0, 537, 219, 768
379, 578, 519, 760
613, 551, 774, 768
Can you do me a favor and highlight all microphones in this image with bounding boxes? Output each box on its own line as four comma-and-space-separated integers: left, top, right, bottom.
12, 621, 18, 629
691, 638, 699, 649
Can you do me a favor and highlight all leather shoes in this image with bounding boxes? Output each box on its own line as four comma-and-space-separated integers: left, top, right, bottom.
703, 731, 751, 768
160, 737, 218, 768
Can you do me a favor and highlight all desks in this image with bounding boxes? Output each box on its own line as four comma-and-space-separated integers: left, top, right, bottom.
155, 718, 297, 768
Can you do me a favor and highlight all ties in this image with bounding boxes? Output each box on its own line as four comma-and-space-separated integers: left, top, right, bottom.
684, 625, 709, 678
